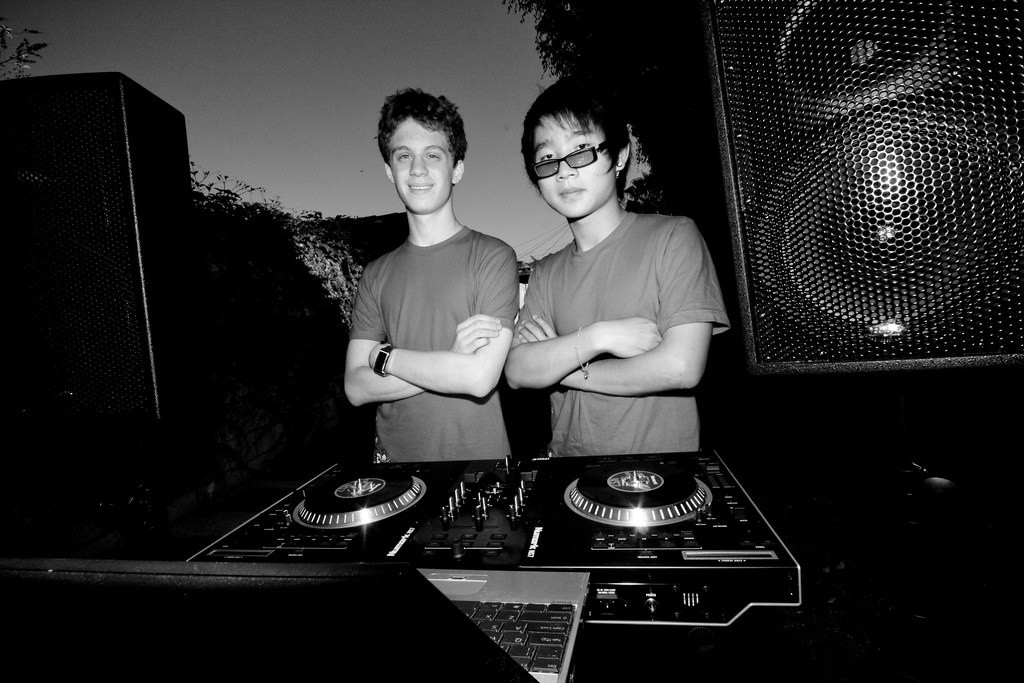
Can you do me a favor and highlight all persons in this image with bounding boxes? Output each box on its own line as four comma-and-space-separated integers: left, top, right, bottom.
505, 71, 730, 455
344, 85, 522, 464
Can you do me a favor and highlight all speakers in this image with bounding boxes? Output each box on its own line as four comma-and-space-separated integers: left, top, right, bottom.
0, 72, 208, 434
700, 0, 1024, 381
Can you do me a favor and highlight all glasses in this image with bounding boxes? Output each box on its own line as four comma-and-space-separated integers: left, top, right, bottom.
533, 141, 607, 179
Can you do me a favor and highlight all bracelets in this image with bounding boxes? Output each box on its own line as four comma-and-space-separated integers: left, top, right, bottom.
373, 345, 398, 376
575, 325, 591, 380
